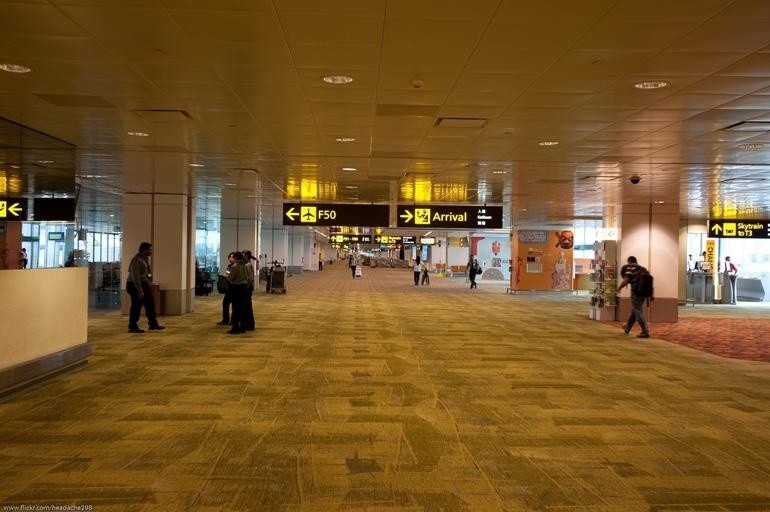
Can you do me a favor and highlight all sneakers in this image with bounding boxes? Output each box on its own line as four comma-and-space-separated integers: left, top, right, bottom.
637, 333, 649, 338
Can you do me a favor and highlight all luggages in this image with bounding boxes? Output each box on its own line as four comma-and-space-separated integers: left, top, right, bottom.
355, 264, 362, 277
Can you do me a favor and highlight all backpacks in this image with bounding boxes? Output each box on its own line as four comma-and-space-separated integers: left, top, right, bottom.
635, 273, 653, 297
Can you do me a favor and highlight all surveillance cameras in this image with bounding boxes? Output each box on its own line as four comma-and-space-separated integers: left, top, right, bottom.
628, 176, 641, 184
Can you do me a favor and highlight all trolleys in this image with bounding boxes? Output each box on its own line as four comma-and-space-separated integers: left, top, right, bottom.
421, 270, 430, 285
265, 265, 287, 294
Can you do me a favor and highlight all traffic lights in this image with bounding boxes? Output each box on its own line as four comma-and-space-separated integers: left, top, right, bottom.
459, 237, 467, 248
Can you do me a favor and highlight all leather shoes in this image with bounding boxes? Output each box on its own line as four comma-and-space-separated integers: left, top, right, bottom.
217, 320, 255, 334
127, 328, 144, 333
148, 326, 165, 330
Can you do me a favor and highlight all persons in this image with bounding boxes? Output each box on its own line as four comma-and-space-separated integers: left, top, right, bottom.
617, 256, 654, 337
702, 251, 707, 261
22, 248, 27, 268
126, 242, 165, 333
414, 256, 427, 285
465, 255, 479, 288
217, 250, 256, 334
319, 253, 322, 270
349, 255, 357, 278
687, 254, 695, 269
726, 256, 738, 274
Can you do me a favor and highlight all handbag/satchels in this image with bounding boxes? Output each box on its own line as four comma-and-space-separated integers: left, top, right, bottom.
217, 276, 227, 294
476, 268, 482, 274
